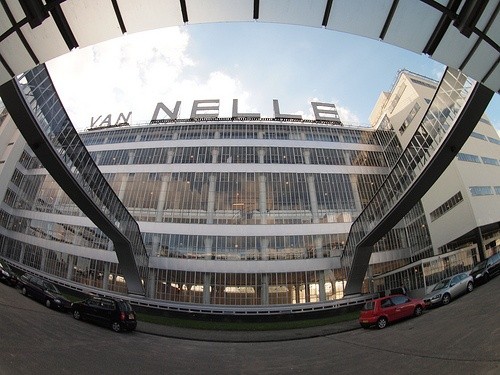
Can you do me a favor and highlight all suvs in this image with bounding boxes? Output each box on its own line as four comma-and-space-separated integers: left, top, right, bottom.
20, 272, 73, 311
72, 296, 137, 332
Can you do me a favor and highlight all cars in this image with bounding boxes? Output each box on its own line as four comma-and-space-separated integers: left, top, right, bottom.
422, 272, 474, 305
469, 252, 500, 282
0, 259, 20, 286
359, 294, 426, 329
